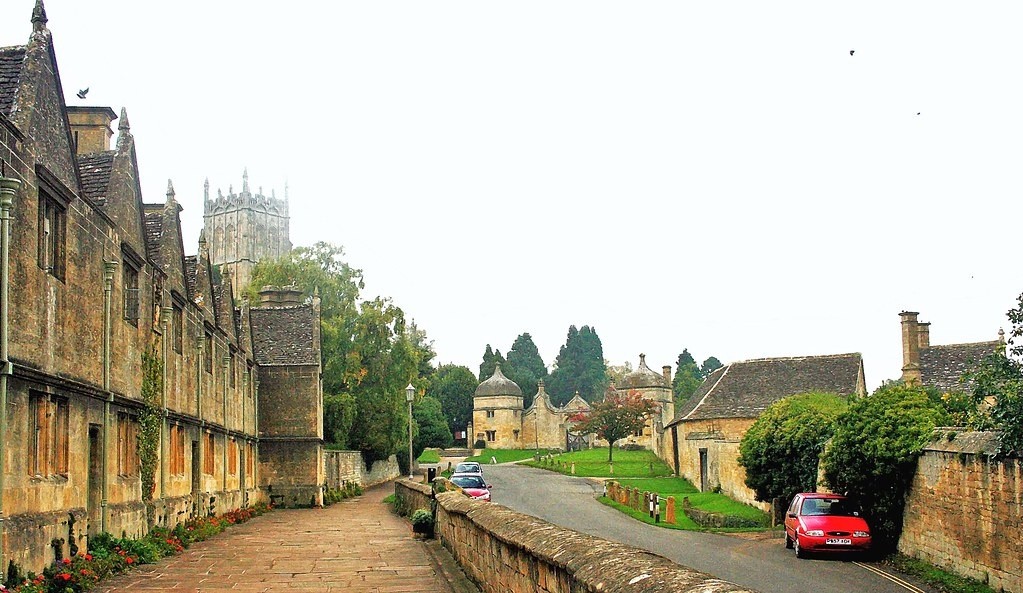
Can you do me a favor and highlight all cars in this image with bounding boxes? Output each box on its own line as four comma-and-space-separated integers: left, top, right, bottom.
448, 462, 493, 502
783, 492, 872, 559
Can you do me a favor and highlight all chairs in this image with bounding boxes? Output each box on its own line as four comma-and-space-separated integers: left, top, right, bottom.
830, 502, 842, 515
804, 500, 816, 513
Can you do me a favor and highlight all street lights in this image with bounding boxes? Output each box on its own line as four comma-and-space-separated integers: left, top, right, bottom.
532, 404, 537, 449
405, 383, 416, 482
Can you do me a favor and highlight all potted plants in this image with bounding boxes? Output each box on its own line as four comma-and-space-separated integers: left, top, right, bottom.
410, 508, 436, 534
